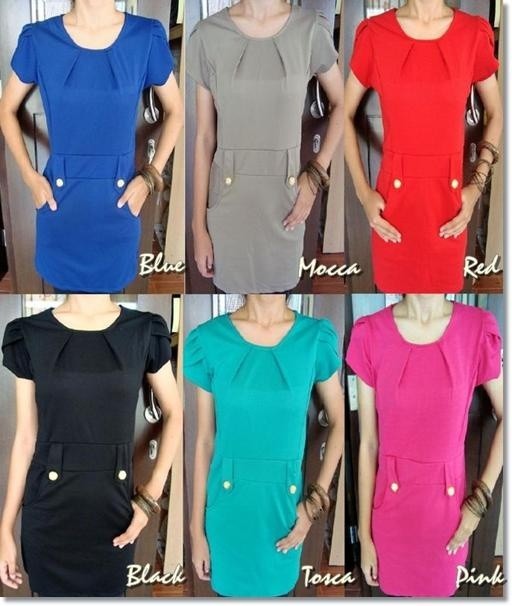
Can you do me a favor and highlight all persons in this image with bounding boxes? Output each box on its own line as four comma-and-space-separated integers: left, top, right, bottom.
345, 293, 504, 599
0, 293, 184, 598
186, 293, 344, 596
189, 0, 344, 294
343, 1, 502, 293
0, 1, 183, 291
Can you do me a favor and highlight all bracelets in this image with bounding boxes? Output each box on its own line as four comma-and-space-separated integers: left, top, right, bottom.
138, 164, 163, 196
470, 139, 499, 194
466, 479, 493, 519
133, 485, 161, 519
306, 159, 331, 195
303, 481, 331, 526
